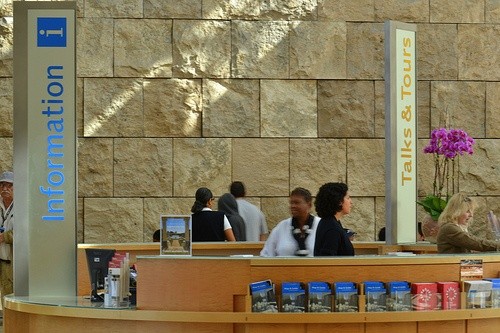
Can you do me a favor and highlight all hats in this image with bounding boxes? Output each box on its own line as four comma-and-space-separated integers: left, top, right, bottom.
0, 172, 14, 184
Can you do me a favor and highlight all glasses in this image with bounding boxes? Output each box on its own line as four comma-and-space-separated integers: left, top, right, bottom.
211, 196, 216, 201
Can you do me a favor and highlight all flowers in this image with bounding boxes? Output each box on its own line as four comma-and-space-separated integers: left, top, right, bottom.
417, 128, 474, 220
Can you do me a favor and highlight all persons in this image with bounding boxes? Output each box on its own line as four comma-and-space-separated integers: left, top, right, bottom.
230, 180, 269, 242
313, 182, 355, 257
0, 169, 13, 309
216, 193, 247, 245
259, 187, 321, 258
378, 222, 426, 241
435, 192, 500, 253
191, 187, 236, 243
152, 230, 160, 242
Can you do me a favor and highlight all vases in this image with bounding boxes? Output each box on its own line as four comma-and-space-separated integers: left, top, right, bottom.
421, 214, 439, 243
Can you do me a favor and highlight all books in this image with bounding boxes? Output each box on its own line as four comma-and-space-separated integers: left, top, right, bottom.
104, 251, 129, 308
249, 278, 500, 313
